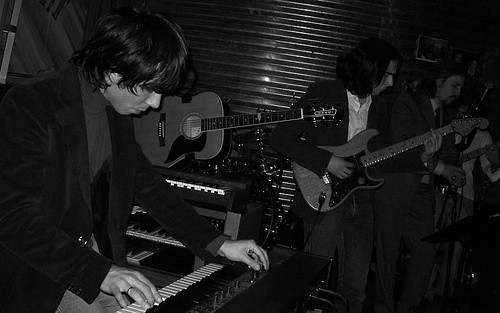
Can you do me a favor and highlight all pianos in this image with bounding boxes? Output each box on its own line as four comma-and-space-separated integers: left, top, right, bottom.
115, 247, 295, 313
124, 172, 233, 271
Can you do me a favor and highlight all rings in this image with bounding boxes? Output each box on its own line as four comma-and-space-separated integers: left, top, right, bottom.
127, 287, 134, 294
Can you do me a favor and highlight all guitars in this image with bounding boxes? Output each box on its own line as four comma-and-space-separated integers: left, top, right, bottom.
132, 92, 336, 168
292, 118, 489, 213
436, 143, 500, 184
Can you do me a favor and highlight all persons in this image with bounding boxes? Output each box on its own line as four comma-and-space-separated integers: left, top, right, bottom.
374, 63, 500, 313
270, 37, 442, 313
1, 8, 269, 313
